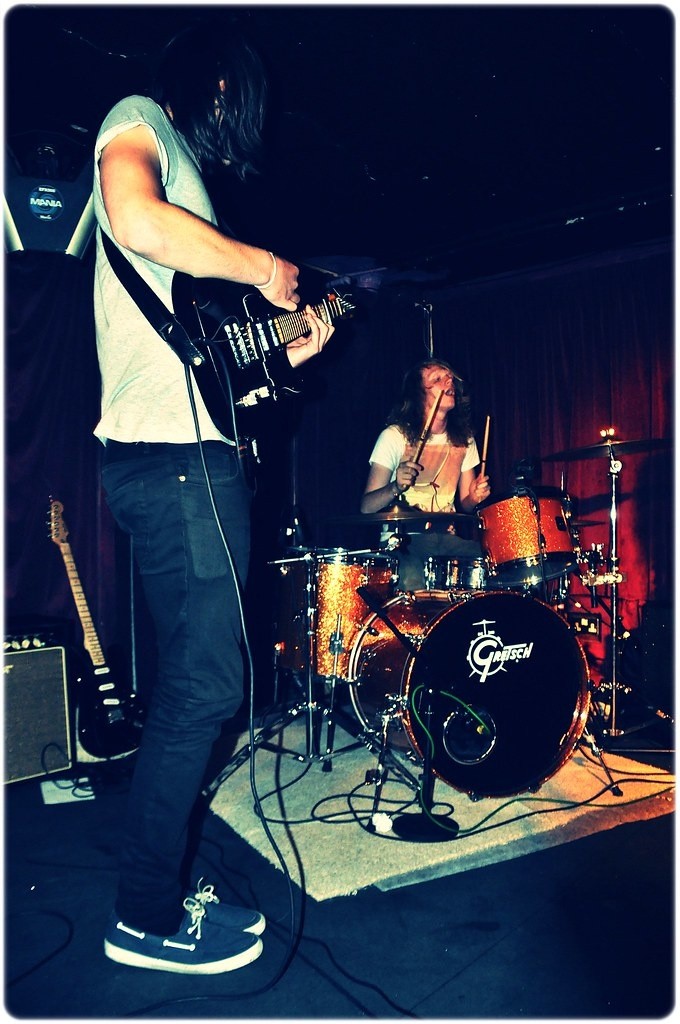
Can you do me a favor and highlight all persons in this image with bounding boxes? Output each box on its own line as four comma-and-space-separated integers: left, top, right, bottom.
360, 360, 491, 594
92, 25, 335, 975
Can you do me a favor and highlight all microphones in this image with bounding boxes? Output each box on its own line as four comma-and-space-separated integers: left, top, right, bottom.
476, 724, 493, 742
570, 520, 603, 527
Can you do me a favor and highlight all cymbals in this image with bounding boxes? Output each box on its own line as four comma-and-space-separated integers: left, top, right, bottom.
312, 496, 477, 531
534, 433, 674, 468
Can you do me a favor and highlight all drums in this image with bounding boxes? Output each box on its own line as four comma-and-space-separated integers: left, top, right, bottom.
475, 478, 583, 588
350, 587, 592, 796
269, 550, 399, 693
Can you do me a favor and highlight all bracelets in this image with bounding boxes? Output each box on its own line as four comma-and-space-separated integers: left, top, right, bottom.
394, 479, 405, 501
254, 250, 277, 289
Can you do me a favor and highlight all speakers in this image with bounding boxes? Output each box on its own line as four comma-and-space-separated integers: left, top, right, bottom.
3, 613, 71, 786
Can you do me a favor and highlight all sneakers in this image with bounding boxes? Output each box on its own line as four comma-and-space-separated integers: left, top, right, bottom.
103, 877, 267, 976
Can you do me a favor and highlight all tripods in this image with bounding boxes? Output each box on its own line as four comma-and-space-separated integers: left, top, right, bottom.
591, 447, 674, 737
201, 522, 419, 802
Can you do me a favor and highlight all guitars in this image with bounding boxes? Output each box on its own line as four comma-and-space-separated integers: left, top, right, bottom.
163, 245, 394, 461
48, 499, 148, 762
